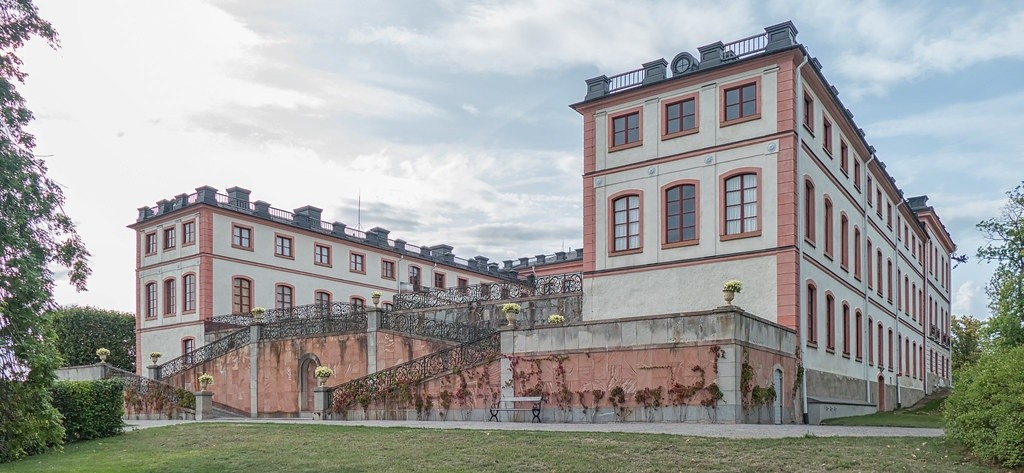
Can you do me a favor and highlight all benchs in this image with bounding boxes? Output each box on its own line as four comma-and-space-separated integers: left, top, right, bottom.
489, 394, 543, 423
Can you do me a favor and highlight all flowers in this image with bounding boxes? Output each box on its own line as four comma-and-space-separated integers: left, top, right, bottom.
95, 347, 111, 356
198, 373, 213, 385
502, 302, 522, 315
251, 307, 265, 314
314, 366, 335, 378
371, 290, 383, 296
548, 315, 565, 323
722, 279, 744, 293
149, 351, 163, 359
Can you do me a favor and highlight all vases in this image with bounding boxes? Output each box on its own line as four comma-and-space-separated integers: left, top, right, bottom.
254, 313, 262, 322
318, 377, 329, 388
152, 357, 158, 365
722, 290, 735, 305
550, 321, 560, 325
201, 381, 208, 391
372, 297, 380, 307
505, 310, 517, 326
100, 355, 106, 363
